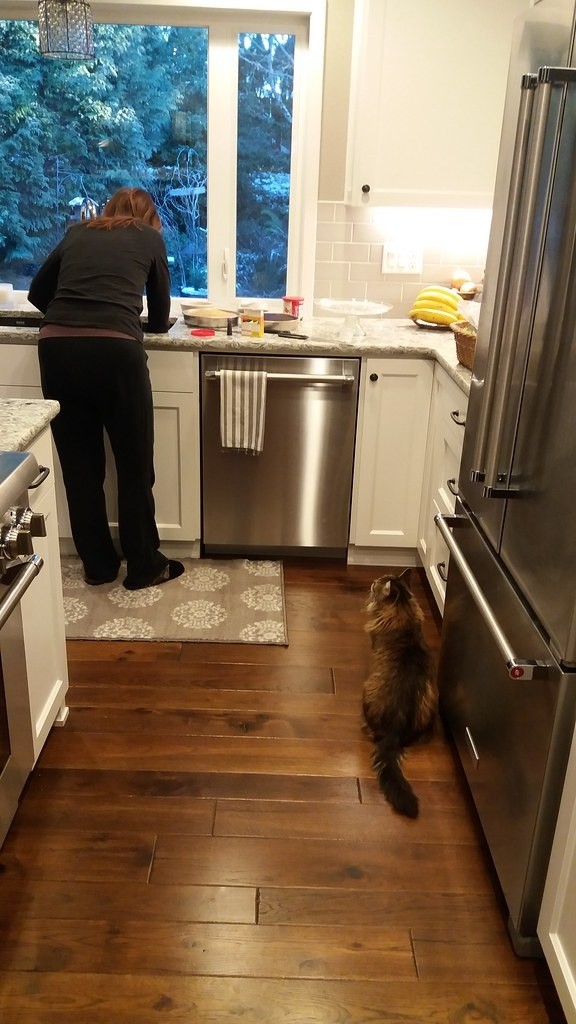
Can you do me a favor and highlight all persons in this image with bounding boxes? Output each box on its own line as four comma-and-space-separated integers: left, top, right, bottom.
28, 189, 184, 592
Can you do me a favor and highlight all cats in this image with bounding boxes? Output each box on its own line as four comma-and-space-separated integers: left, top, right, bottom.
362, 566, 437, 821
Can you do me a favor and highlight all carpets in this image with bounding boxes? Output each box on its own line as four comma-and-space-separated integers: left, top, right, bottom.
61, 554, 289, 646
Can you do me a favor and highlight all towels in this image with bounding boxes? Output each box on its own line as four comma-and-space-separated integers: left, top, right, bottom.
219, 369, 269, 457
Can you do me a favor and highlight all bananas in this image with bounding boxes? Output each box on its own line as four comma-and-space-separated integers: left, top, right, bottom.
408, 285, 463, 326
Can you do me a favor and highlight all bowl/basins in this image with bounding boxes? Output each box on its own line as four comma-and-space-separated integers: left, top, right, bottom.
183, 301, 213, 309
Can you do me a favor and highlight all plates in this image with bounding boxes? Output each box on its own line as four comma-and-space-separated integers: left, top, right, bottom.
180, 309, 238, 326
454, 292, 475, 299
243, 314, 300, 332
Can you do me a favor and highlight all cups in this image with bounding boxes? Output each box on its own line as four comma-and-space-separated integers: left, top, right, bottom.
0, 284, 12, 307
13, 290, 29, 305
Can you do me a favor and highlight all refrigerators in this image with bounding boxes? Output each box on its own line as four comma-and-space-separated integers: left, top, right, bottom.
435, 0, 576, 956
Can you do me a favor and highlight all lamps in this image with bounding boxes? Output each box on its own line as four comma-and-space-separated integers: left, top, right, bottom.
37, 1, 95, 60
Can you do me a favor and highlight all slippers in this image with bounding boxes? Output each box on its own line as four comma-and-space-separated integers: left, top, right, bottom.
83, 556, 185, 591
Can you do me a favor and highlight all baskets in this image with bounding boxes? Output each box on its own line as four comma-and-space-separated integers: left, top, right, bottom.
449, 320, 477, 371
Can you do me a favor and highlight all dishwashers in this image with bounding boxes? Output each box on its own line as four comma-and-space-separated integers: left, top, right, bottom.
199, 352, 359, 563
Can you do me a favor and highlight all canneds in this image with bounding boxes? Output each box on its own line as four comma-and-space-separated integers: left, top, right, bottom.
283, 296, 304, 321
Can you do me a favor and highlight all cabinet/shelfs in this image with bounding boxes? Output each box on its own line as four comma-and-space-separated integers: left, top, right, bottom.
347, 357, 468, 617
0, 424, 70, 852
0, 345, 201, 559
